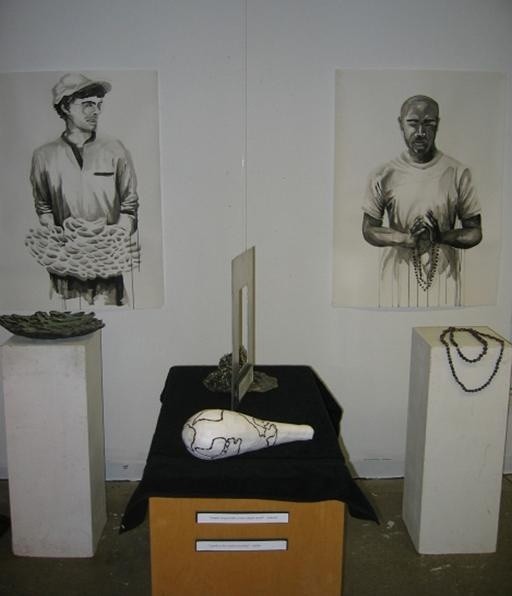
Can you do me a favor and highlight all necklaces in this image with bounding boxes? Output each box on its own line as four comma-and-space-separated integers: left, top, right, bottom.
440, 327, 504, 392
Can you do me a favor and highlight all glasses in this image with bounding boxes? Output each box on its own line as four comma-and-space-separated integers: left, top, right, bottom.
70, 101, 102, 115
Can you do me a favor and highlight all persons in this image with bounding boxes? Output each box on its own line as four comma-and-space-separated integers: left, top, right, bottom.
30, 73, 139, 308
362, 95, 482, 309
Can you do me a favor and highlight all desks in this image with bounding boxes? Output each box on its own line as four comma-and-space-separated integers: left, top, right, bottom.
142, 362, 346, 595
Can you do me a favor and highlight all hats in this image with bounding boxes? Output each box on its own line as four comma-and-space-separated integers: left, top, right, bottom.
51, 71, 113, 107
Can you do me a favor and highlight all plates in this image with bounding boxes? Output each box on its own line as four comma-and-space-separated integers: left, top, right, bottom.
1, 311, 104, 339
